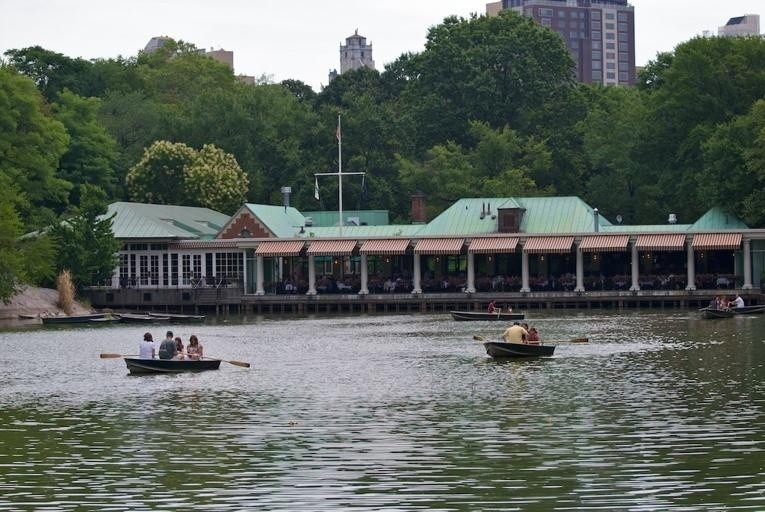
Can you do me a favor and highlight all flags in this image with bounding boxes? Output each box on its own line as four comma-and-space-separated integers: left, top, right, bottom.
336, 122, 342, 140
362, 175, 371, 198
314, 178, 320, 200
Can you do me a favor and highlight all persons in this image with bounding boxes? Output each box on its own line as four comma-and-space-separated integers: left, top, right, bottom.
488, 298, 498, 313
508, 304, 513, 313
503, 322, 528, 343
720, 295, 729, 308
174, 336, 185, 360
283, 272, 738, 294
185, 335, 203, 360
521, 323, 529, 343
733, 293, 745, 307
158, 331, 184, 361
528, 327, 540, 344
139, 331, 156, 358
711, 296, 721, 310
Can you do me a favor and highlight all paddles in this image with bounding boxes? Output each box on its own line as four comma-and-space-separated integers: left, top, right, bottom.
473, 335, 497, 342
528, 339, 588, 342
179, 352, 251, 368
101, 354, 140, 358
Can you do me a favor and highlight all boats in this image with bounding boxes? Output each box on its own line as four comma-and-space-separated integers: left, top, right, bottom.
699, 308, 736, 320
449, 310, 525, 321
483, 341, 556, 357
37, 309, 207, 333
729, 305, 764, 314
124, 356, 221, 375
